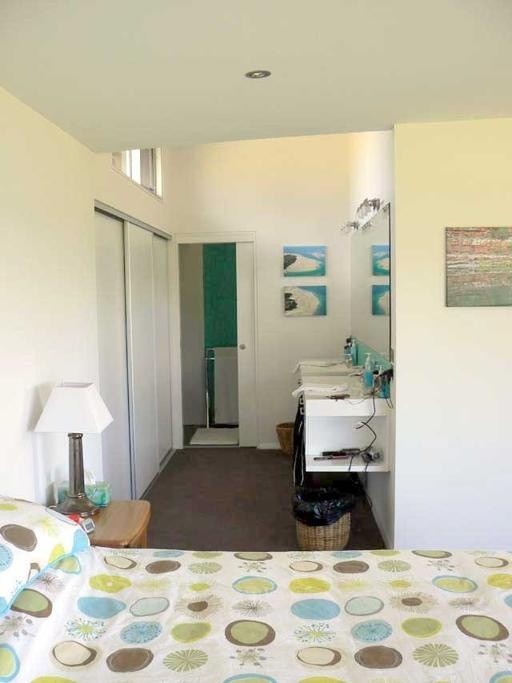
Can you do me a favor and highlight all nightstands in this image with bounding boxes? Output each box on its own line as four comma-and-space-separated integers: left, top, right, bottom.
63, 500, 152, 549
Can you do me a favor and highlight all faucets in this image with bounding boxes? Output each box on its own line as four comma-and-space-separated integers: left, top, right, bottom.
341, 354, 354, 367
349, 372, 363, 377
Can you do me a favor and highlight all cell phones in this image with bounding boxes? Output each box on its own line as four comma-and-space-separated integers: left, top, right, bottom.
314, 448, 360, 461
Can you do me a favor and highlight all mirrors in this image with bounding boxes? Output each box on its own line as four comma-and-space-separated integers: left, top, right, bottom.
349, 203, 392, 363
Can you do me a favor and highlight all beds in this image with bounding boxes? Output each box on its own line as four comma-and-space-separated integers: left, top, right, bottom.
0, 544, 512, 683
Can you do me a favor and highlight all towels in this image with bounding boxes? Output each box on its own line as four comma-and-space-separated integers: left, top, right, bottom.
291, 385, 348, 395
292, 360, 330, 374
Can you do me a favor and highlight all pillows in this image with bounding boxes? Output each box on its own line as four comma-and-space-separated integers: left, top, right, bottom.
0, 494, 92, 619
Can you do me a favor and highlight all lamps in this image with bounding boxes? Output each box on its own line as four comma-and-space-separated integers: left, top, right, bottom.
33, 381, 114, 517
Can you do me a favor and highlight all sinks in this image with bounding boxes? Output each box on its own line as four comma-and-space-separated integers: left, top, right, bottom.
311, 378, 359, 392
312, 362, 344, 372
